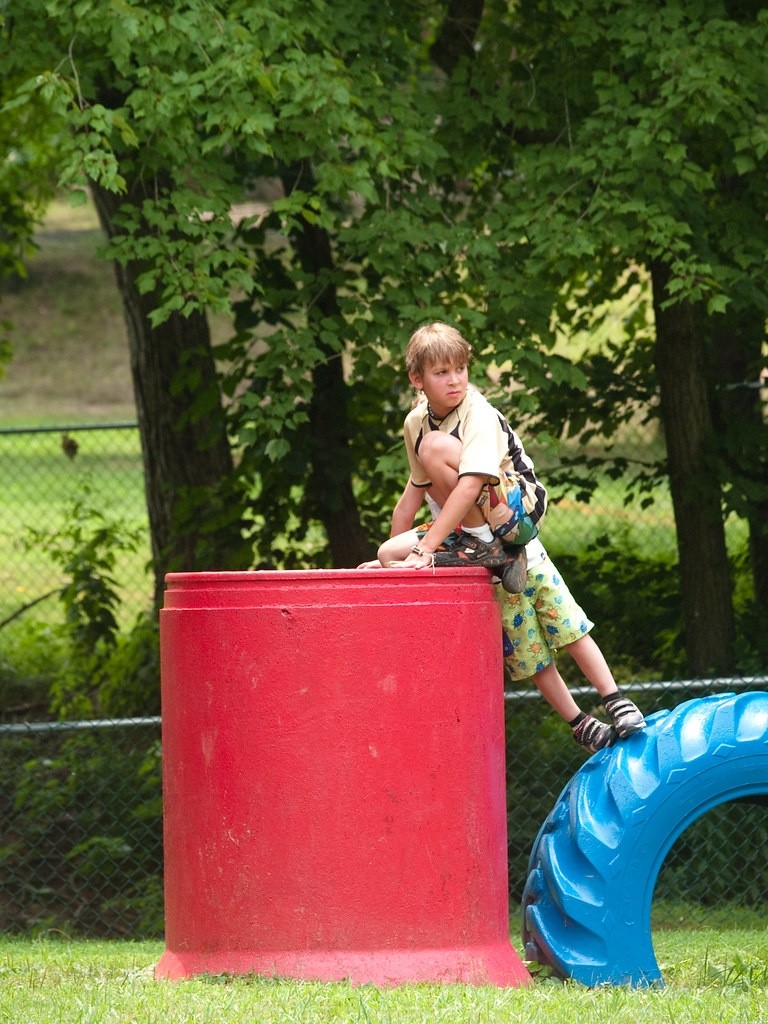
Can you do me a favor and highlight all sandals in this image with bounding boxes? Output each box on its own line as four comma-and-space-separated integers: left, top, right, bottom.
432, 529, 506, 566
490, 544, 527, 594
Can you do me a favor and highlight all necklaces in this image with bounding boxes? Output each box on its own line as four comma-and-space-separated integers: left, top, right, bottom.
428, 404, 439, 418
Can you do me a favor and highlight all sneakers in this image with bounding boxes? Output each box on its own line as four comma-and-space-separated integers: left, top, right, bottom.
572, 715, 617, 754
604, 696, 646, 738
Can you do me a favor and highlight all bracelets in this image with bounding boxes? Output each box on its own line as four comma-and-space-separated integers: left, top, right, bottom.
411, 547, 436, 575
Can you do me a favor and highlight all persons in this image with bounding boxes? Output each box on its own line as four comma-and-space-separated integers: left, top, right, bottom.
357, 323, 546, 593
424, 492, 647, 752
